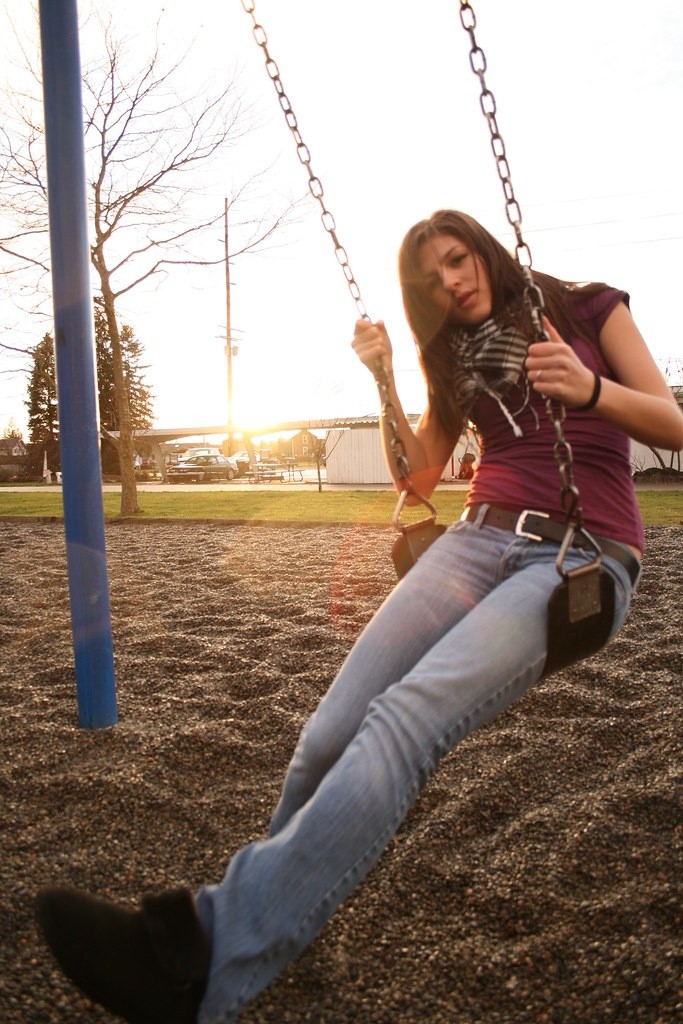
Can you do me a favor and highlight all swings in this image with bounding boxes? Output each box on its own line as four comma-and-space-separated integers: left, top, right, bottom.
243, 0, 620, 678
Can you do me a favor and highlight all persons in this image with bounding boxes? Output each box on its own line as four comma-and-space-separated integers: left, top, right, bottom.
36, 210, 683, 1024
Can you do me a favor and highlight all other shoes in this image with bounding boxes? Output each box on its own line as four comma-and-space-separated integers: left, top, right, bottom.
35, 885, 210, 1024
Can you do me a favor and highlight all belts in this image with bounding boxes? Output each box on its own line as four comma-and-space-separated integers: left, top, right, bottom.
461, 505, 640, 587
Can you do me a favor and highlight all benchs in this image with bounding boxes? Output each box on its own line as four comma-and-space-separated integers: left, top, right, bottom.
164, 472, 222, 484
245, 470, 304, 484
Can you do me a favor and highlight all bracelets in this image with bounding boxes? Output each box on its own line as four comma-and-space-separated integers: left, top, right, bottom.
574, 374, 601, 413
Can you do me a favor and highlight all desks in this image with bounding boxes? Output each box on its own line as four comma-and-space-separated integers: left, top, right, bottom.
170, 466, 212, 484
252, 463, 298, 484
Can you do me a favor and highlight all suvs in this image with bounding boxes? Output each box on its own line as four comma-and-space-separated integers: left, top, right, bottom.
178, 448, 220, 464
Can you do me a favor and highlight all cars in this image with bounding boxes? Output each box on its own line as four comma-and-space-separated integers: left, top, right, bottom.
166, 454, 238, 483
232, 451, 260, 461
133, 461, 157, 469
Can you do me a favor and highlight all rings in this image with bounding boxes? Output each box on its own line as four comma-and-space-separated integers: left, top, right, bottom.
536, 370, 543, 382
